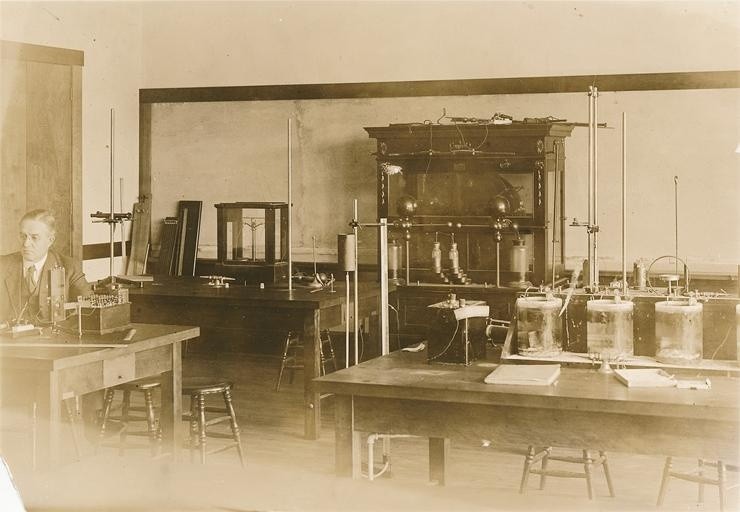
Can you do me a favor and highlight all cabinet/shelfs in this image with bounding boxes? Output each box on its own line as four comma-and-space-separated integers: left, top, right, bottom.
363, 123, 579, 336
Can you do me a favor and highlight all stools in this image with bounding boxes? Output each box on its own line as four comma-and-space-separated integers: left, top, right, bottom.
520, 445, 616, 501
656, 454, 740, 512
276, 329, 338, 392
155, 375, 246, 469
94, 377, 162, 450
31, 398, 81, 470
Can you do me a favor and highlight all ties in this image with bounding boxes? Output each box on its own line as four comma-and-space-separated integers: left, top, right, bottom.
26, 266, 37, 294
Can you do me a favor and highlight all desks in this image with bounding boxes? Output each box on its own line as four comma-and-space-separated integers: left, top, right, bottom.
0, 322, 201, 464
88, 274, 399, 440
309, 338, 740, 487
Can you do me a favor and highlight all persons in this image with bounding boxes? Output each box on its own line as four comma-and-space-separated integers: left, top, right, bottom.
0, 210, 94, 326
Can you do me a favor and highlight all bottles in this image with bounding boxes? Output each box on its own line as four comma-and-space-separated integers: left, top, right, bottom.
653, 299, 705, 367
431, 241, 443, 274
388, 238, 403, 278
447, 242, 460, 275
514, 296, 564, 358
632, 262, 647, 291
508, 238, 528, 283
585, 298, 636, 360
